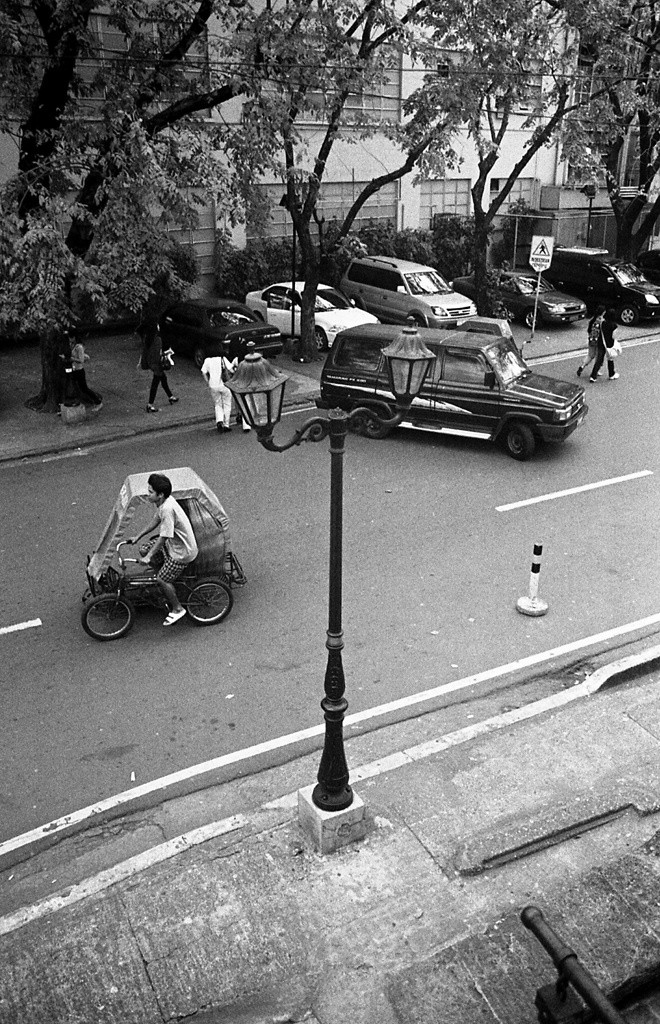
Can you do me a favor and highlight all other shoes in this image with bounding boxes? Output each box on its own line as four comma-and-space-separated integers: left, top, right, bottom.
244, 429, 250, 432
91, 402, 103, 413
596, 372, 602, 376
609, 373, 619, 379
217, 422, 233, 432
577, 367, 583, 377
589, 377, 598, 383
236, 416, 242, 426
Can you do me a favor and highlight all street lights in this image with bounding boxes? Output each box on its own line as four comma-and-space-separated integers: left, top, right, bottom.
582, 185, 595, 248
225, 317, 437, 855
279, 194, 296, 354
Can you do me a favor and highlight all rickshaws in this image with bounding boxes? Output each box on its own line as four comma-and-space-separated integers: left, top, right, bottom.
81, 466, 246, 642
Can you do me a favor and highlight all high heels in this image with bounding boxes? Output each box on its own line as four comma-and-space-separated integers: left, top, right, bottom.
146, 404, 158, 413
169, 397, 179, 405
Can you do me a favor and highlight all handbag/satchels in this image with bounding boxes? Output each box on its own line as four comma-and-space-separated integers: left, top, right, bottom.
161, 351, 171, 370
220, 356, 230, 384
606, 340, 622, 361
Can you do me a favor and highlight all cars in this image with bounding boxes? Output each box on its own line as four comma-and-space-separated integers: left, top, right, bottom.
161, 298, 283, 363
453, 271, 587, 330
245, 282, 382, 352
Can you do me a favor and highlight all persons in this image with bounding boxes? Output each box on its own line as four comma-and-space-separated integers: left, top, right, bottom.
137, 326, 179, 413
126, 473, 199, 626
56, 335, 104, 416
576, 305, 620, 383
201, 341, 251, 432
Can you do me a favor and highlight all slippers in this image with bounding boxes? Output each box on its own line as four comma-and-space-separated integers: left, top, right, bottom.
163, 609, 186, 625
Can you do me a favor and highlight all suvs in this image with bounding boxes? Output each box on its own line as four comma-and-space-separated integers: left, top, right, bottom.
340, 256, 478, 330
315, 323, 589, 461
542, 246, 660, 326
